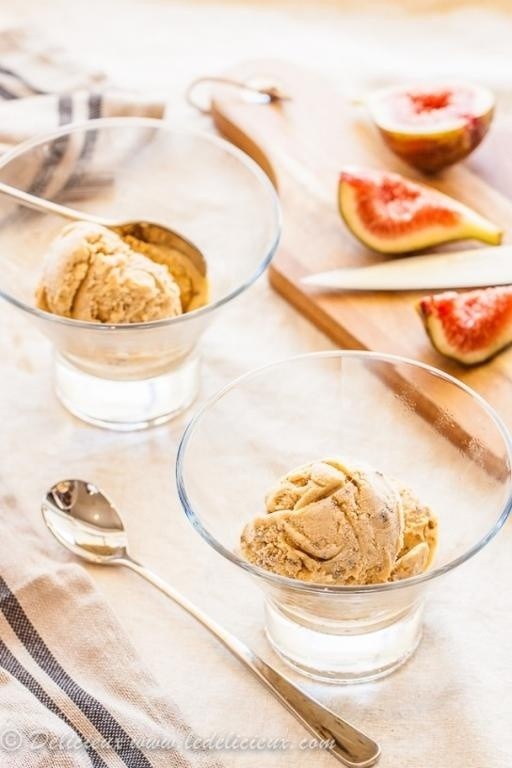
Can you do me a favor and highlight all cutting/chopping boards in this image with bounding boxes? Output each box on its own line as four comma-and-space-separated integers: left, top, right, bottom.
217, 69, 512, 477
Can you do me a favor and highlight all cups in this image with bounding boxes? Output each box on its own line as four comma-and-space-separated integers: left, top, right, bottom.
2, 117, 281, 429
176, 352, 512, 687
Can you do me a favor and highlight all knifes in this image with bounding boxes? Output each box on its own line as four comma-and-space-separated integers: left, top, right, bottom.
297, 243, 512, 299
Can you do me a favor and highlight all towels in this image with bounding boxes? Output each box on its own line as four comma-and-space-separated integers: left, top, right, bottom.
0, 487, 222, 766
1, 26, 167, 235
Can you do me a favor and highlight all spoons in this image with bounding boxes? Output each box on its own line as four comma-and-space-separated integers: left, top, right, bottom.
41, 480, 384, 768
1, 179, 209, 285
